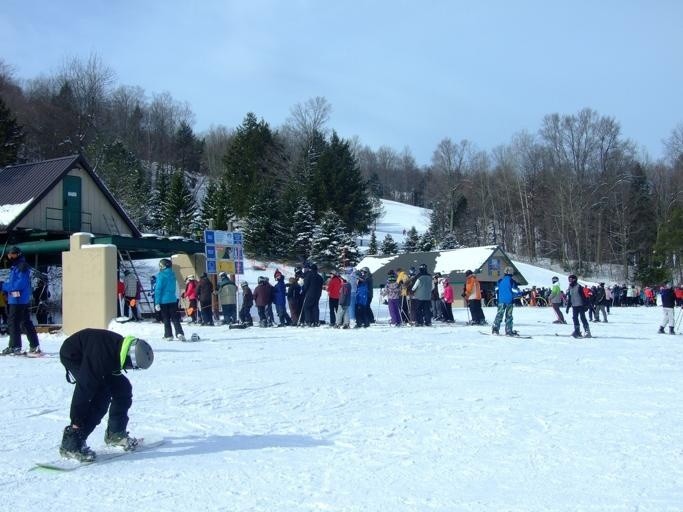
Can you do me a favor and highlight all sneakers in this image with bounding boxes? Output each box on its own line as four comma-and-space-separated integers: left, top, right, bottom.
104, 428, 139, 448
59, 441, 98, 465
257, 318, 376, 329
570, 331, 579, 337
467, 320, 477, 323
479, 318, 487, 324
0, 346, 21, 355
163, 335, 174, 340
179, 334, 187, 340
584, 330, 591, 337
491, 328, 499, 334
27, 346, 41, 355
389, 315, 455, 327
185, 318, 255, 325
506, 331, 519, 336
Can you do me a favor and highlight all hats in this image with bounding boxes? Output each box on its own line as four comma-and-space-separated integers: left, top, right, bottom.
279, 263, 369, 282
552, 276, 558, 283
388, 264, 428, 273
465, 270, 473, 276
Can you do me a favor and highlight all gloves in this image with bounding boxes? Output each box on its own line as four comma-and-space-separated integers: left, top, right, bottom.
566, 307, 570, 312
155, 303, 160, 312
61, 426, 85, 451
584, 304, 590, 311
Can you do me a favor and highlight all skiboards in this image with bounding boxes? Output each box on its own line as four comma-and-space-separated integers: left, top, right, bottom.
29, 439, 167, 472
556, 333, 599, 338
479, 331, 530, 339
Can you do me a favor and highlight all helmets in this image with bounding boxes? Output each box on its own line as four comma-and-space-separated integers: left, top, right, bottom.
7, 248, 22, 267
503, 266, 514, 274
158, 259, 170, 266
130, 337, 154, 369
569, 275, 577, 285
185, 272, 268, 285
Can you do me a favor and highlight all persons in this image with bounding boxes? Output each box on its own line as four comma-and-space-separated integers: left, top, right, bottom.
566, 275, 613, 338
380, 265, 457, 328
514, 282, 682, 312
0, 245, 46, 359
130, 273, 145, 322
57, 327, 154, 465
0, 281, 12, 326
492, 267, 521, 336
463, 269, 488, 327
550, 276, 567, 324
146, 276, 162, 324
657, 282, 677, 335
182, 264, 376, 328
118, 270, 139, 322
153, 259, 186, 342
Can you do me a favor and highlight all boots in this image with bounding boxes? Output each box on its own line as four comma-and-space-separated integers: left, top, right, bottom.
668, 326, 675, 334
657, 326, 665, 332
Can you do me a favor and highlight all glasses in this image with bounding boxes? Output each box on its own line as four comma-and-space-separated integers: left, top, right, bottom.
6, 252, 21, 260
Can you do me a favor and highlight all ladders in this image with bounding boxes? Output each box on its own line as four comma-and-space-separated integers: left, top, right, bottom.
103, 214, 154, 318
